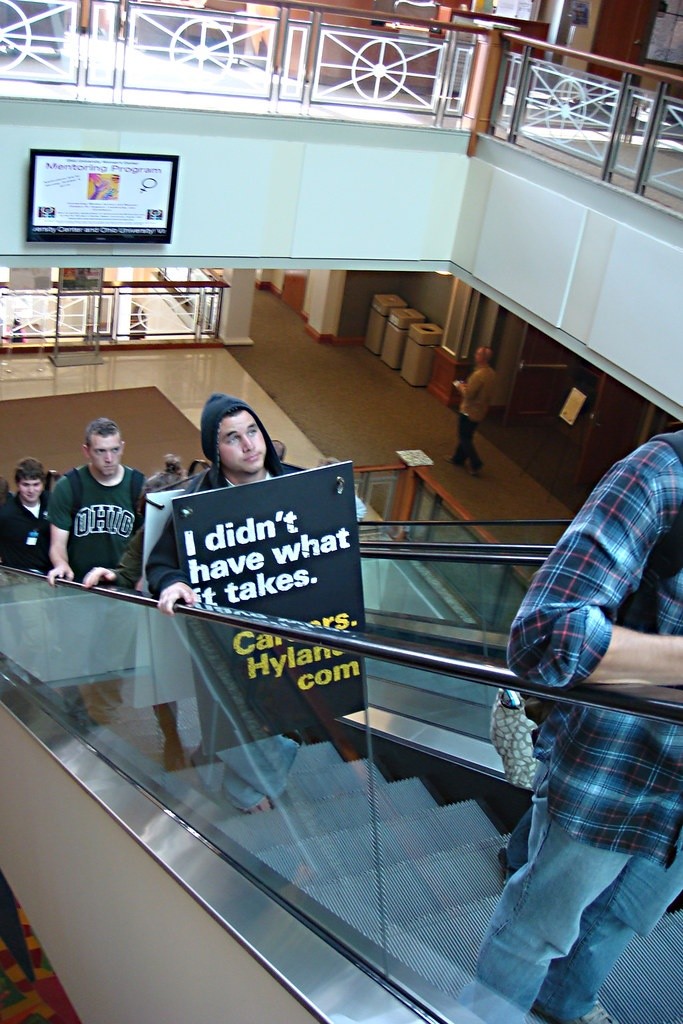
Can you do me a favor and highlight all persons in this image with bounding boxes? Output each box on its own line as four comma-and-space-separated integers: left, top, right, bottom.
444, 347, 497, 474
144, 392, 366, 815
0, 458, 54, 678
83, 454, 222, 773
47, 418, 144, 758
11, 319, 27, 344
473, 430, 683, 1024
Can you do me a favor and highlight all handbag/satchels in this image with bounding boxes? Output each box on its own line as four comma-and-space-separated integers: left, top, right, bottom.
490, 688, 542, 789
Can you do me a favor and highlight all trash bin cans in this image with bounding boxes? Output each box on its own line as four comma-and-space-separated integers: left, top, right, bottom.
401, 322, 444, 387
364, 294, 426, 370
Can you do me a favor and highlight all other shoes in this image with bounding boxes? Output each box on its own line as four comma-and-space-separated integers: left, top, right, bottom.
444, 455, 464, 466
249, 796, 272, 813
464, 464, 478, 476
530, 997, 618, 1024
498, 847, 516, 883
